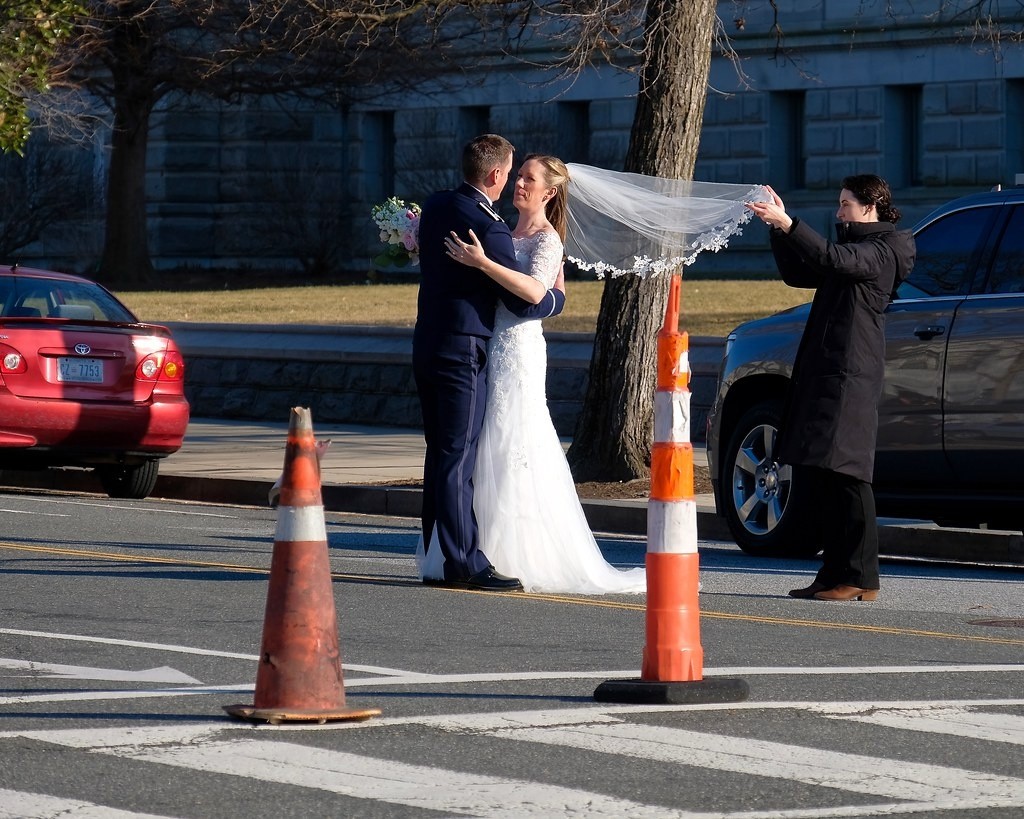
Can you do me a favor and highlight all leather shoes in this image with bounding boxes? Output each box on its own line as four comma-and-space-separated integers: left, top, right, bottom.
447, 564, 522, 592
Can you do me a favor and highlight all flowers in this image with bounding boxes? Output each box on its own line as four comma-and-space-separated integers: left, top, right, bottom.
370, 195, 422, 269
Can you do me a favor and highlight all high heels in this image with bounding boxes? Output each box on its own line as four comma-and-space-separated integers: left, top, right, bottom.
814, 584, 878, 601
789, 580, 833, 599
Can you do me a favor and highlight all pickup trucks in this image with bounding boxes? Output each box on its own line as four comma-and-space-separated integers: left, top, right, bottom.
709, 182, 1024, 559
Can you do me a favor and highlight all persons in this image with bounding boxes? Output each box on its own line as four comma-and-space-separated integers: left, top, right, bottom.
444, 154, 569, 596
745, 175, 918, 602
412, 135, 566, 590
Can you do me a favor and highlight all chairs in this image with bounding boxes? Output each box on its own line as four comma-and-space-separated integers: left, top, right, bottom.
46, 305, 96, 320
7, 307, 41, 317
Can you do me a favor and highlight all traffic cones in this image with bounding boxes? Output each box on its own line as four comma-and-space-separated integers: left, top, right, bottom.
222, 404, 386, 724
591, 275, 753, 705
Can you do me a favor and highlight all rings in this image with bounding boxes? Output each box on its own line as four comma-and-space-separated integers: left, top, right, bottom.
452, 250, 456, 254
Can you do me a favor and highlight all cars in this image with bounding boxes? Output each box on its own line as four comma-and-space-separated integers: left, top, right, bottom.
0, 262, 189, 501
